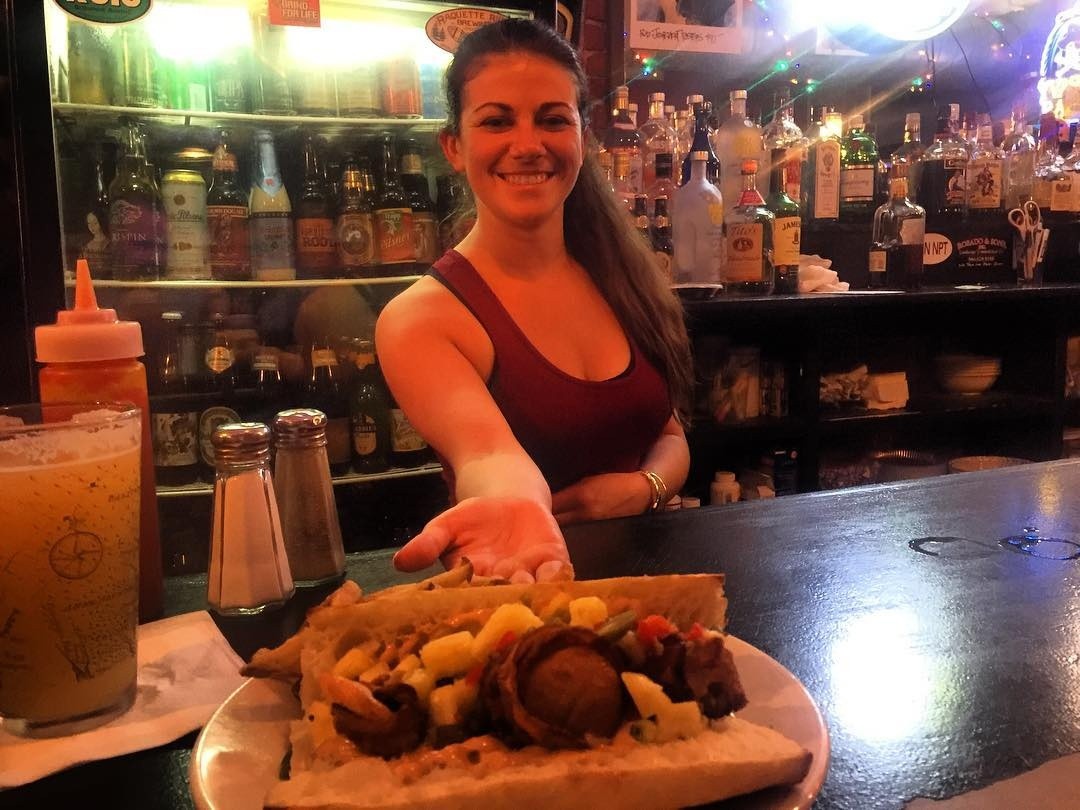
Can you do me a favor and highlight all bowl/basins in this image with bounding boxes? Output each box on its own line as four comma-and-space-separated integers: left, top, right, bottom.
869, 448, 1035, 482
935, 356, 1001, 395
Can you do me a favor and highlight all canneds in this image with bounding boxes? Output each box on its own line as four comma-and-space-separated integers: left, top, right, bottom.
161, 169, 212, 281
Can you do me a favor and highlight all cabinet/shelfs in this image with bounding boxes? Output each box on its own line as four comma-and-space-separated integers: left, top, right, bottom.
44, 0, 534, 498
675, 280, 1080, 511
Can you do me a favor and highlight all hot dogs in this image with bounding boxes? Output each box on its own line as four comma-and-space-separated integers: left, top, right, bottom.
261, 569, 816, 810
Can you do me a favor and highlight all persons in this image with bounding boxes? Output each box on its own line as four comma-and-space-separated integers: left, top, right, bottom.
369, 17, 691, 584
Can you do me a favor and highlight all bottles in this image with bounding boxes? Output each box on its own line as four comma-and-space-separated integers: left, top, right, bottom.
873, 164, 926, 290
65, 117, 164, 282
674, 90, 769, 210
926, 104, 1002, 216
1002, 98, 1080, 212
592, 87, 676, 197
148, 309, 299, 485
295, 131, 477, 278
672, 150, 724, 285
764, 86, 888, 222
629, 194, 672, 275
888, 112, 929, 206
208, 123, 297, 283
273, 409, 346, 588
305, 339, 434, 478
208, 423, 296, 619
724, 161, 800, 295
664, 472, 741, 510
34, 260, 163, 622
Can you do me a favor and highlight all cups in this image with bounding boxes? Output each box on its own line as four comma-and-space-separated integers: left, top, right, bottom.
1015, 230, 1048, 286
1, 401, 142, 739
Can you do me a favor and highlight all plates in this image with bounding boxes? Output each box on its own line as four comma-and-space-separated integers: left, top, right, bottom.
191, 633, 831, 810
670, 284, 723, 301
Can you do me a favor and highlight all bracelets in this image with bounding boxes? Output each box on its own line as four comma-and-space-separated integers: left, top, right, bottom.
641, 468, 667, 513
